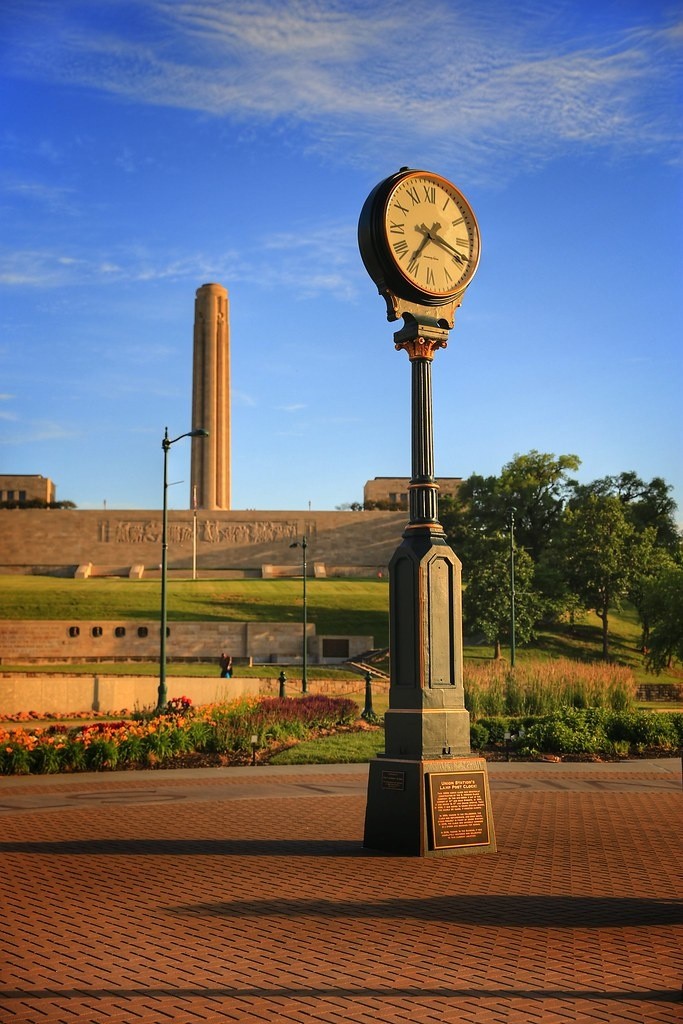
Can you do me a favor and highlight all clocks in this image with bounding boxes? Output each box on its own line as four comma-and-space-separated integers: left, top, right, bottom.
358, 166, 482, 328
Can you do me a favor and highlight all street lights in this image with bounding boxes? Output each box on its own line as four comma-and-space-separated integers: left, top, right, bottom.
290, 537, 308, 694
157, 423, 208, 710
501, 506, 515, 667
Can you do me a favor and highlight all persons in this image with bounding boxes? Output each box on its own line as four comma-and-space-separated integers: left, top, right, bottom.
220, 652, 233, 678
